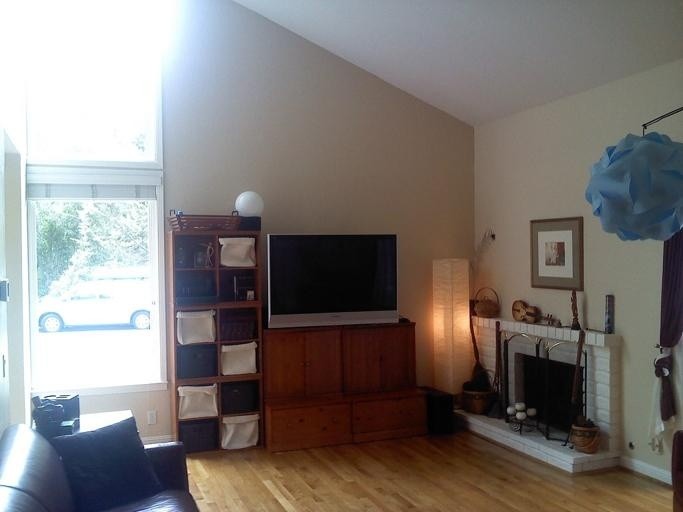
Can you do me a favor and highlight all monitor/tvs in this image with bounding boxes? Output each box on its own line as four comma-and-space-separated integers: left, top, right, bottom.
267, 233, 400, 329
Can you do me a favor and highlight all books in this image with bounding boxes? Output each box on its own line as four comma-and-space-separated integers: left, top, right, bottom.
604, 294, 614, 334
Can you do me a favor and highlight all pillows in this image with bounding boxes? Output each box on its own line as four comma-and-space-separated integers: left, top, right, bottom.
48, 415, 164, 512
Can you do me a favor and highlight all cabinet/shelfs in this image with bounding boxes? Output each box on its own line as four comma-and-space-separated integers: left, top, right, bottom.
164, 229, 266, 454
262, 313, 427, 453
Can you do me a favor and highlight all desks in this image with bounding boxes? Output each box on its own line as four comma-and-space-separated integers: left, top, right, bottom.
32, 410, 134, 435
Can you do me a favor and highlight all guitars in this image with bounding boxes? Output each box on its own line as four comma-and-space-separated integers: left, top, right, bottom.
512, 300, 561, 328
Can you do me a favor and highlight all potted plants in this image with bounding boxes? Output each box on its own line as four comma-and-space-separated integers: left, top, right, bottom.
568, 415, 601, 453
461, 378, 493, 416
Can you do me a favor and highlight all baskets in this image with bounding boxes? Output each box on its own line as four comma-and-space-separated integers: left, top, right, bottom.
573, 423, 600, 455
464, 384, 494, 415
167, 208, 241, 231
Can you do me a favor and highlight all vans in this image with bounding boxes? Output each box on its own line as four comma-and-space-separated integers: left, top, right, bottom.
37, 277, 151, 333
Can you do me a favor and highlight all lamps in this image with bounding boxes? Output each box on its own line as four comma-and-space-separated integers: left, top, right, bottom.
506, 400, 536, 435
431, 257, 475, 395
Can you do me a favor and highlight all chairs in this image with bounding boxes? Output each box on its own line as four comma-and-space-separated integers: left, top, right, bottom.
0, 422, 200, 512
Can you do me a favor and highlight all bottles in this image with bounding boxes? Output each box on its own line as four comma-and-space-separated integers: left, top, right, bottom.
526, 306, 537, 323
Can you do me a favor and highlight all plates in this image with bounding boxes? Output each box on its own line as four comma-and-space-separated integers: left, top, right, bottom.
511, 300, 525, 320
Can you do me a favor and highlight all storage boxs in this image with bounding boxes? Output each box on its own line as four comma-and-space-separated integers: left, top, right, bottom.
221, 380, 260, 414
178, 418, 219, 454
175, 270, 218, 305
177, 343, 218, 379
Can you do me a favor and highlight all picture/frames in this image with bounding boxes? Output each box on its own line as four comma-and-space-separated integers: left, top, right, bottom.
529, 216, 584, 291
